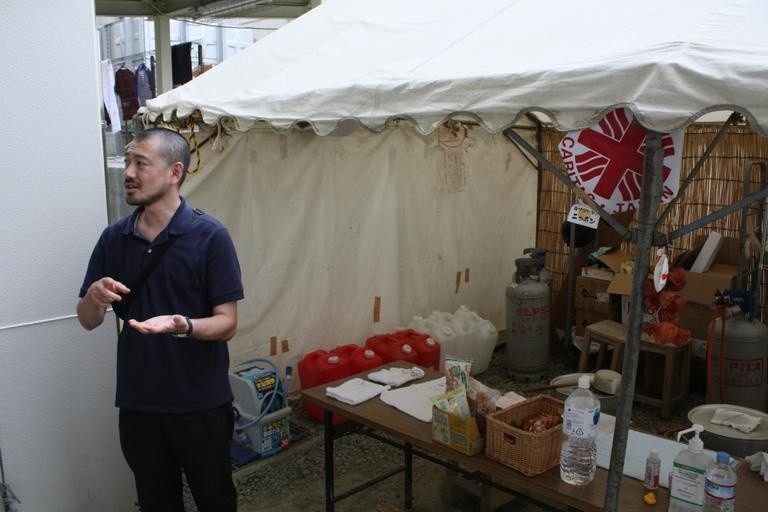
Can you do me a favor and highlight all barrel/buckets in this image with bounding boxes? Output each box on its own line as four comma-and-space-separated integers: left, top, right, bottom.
296, 305, 498, 426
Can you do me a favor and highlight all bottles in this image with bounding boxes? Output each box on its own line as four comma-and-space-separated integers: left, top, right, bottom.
559, 376, 601, 487
643, 448, 661, 490
701, 451, 738, 512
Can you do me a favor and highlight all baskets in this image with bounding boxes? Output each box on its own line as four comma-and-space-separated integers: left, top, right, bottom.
485, 393, 565, 477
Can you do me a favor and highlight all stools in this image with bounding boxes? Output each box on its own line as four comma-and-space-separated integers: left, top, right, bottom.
578, 319, 692, 420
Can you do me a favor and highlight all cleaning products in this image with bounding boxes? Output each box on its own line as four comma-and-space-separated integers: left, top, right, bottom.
668, 424, 714, 512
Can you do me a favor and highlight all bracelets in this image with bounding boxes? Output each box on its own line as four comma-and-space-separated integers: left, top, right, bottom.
166, 312, 193, 339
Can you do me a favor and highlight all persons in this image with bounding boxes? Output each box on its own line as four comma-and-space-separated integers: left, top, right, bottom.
71, 126, 246, 512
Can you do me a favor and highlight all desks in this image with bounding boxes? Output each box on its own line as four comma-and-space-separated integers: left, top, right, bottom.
299, 361, 768, 511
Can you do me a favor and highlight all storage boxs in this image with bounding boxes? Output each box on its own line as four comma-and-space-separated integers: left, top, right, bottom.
573, 234, 739, 402
432, 395, 503, 457
